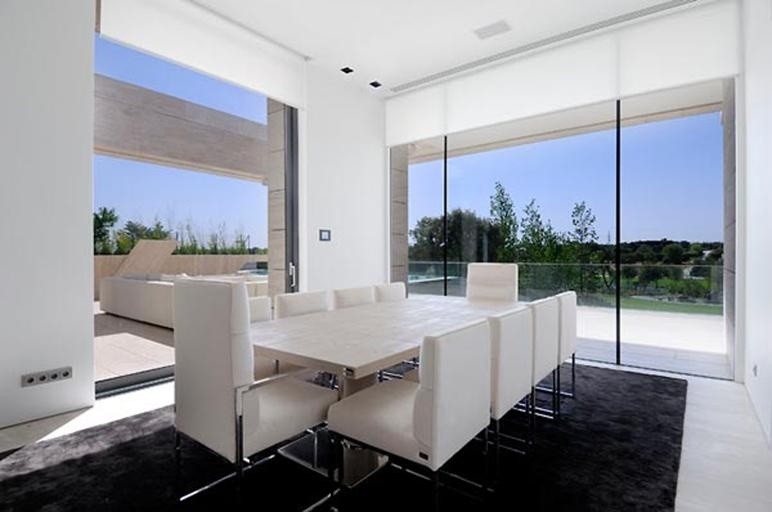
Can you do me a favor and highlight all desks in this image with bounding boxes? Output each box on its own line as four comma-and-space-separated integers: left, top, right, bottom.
249, 298, 529, 490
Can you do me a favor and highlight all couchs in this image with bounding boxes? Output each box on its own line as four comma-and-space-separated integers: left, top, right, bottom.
100, 274, 174, 330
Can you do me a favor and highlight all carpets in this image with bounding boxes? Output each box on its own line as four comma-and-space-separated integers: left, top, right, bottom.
0, 361, 687, 511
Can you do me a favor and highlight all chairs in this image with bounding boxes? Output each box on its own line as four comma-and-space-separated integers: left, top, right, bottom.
332, 287, 375, 312
466, 261, 518, 303
471, 295, 561, 458
172, 274, 341, 511
404, 304, 533, 496
246, 294, 277, 381
272, 290, 327, 321
374, 280, 408, 303
324, 317, 494, 510
512, 289, 580, 422
